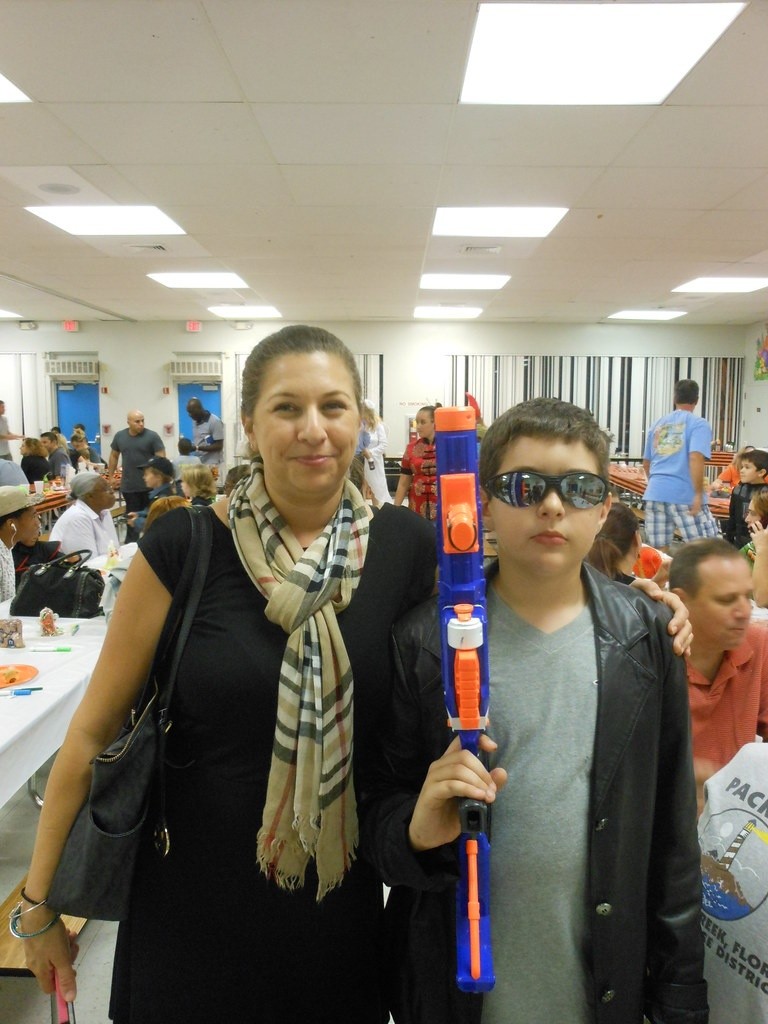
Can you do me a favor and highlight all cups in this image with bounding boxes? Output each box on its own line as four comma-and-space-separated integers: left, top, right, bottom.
20, 484, 30, 495
34, 481, 44, 494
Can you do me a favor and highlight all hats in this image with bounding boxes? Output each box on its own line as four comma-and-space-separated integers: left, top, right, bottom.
137, 456, 175, 477
0, 485, 45, 516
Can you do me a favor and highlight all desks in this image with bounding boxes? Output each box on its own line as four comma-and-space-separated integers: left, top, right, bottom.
609, 451, 737, 518
30, 478, 123, 533
0, 542, 139, 807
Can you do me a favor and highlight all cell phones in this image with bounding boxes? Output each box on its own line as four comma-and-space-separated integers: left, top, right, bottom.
750, 516, 768, 534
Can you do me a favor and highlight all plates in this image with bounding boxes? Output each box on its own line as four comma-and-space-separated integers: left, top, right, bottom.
0, 664, 39, 689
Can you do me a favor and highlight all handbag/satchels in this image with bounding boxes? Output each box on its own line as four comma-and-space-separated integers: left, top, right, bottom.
49, 506, 212, 922
9, 549, 104, 619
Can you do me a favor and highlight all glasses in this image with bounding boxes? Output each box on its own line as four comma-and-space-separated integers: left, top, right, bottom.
486, 471, 612, 507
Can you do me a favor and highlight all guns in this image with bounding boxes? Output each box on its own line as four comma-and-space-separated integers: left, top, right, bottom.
432, 406, 498, 995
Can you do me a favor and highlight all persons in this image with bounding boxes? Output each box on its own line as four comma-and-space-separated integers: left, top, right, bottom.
0, 378, 768, 814
21, 326, 696, 1024
356, 397, 713, 1024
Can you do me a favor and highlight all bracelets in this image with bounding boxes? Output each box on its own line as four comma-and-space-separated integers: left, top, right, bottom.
8, 886, 60, 938
196, 446, 198, 452
